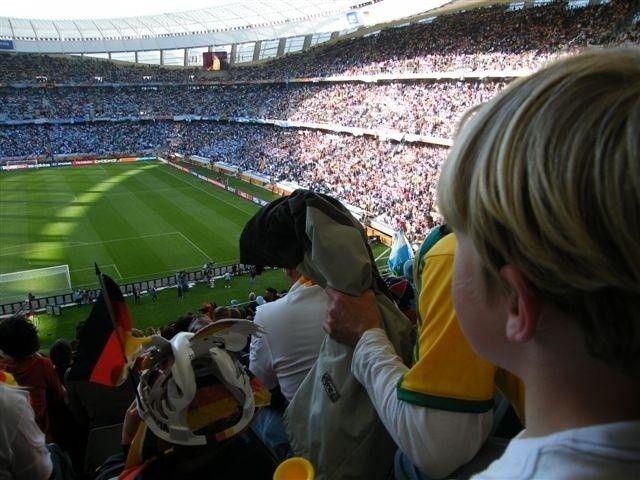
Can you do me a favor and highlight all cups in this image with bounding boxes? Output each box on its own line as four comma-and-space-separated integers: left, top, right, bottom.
274, 457, 315, 480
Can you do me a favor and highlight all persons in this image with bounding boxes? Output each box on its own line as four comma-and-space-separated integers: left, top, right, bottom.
0, 0, 639, 480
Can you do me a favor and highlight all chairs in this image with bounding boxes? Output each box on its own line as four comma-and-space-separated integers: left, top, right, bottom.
83, 422, 123, 475
